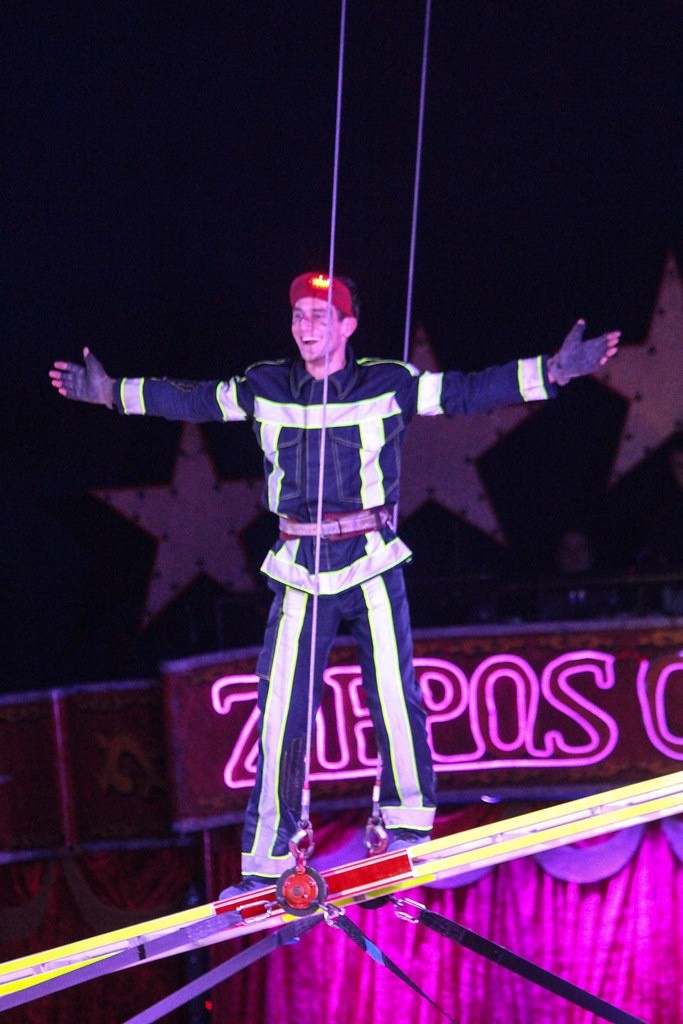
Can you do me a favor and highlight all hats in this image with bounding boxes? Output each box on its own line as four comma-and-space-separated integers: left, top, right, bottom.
289, 273, 359, 321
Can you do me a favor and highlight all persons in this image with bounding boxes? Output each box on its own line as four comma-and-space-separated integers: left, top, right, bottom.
48, 274, 620, 902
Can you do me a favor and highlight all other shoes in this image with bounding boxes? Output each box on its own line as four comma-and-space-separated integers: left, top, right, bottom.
387, 828, 429, 853
219, 879, 274, 903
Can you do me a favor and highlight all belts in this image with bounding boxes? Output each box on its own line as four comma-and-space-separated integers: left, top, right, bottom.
278, 509, 390, 539
